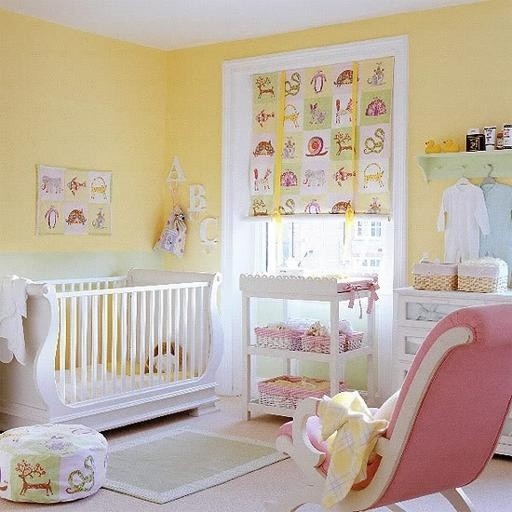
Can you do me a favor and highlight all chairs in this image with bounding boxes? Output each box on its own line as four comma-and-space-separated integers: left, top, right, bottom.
276, 306, 511, 511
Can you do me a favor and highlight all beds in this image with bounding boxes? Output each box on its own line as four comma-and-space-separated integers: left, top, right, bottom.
0, 263, 228, 433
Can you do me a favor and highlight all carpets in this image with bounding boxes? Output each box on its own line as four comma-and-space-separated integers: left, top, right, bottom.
100, 425, 290, 505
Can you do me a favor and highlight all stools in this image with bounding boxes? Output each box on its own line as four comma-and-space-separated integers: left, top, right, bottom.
0, 422, 110, 505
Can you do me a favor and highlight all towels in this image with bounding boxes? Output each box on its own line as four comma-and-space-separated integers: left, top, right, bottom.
317, 388, 390, 508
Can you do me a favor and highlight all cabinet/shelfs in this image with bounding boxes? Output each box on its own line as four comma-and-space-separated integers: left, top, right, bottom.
393, 284, 512, 458
238, 267, 380, 424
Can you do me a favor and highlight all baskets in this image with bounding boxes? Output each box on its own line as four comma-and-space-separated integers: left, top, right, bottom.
254, 327, 364, 354
413, 259, 508, 292
258, 376, 347, 410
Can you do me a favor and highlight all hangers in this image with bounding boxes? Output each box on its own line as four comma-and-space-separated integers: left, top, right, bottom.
454, 161, 496, 188
